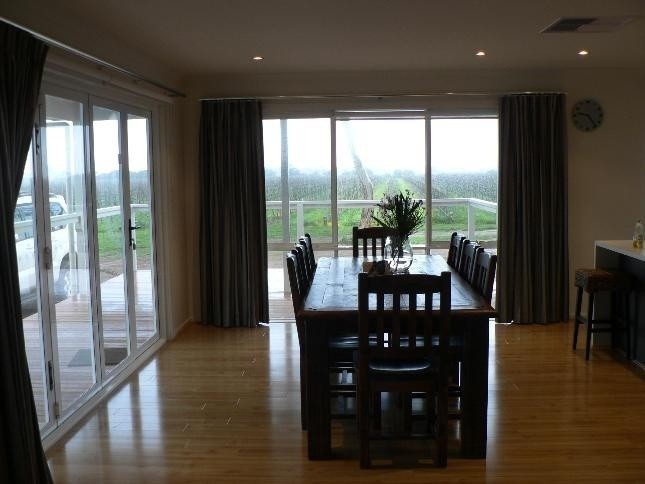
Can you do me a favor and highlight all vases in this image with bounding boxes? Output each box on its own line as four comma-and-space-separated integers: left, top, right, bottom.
385, 237, 413, 273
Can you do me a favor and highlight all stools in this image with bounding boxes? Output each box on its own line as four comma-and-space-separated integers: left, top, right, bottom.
573, 268, 633, 362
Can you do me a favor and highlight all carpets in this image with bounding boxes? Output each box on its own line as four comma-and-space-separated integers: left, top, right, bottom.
66, 347, 128, 367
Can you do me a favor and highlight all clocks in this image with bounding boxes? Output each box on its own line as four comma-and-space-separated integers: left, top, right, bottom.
570, 97, 603, 132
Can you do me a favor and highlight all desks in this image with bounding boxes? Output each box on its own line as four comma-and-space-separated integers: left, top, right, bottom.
302, 255, 497, 458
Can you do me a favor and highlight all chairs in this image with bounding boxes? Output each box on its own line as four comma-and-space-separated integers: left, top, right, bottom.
357, 271, 453, 467
285, 233, 382, 433
401, 231, 497, 433
353, 226, 390, 257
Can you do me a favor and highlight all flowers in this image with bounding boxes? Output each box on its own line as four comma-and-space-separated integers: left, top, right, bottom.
374, 190, 427, 258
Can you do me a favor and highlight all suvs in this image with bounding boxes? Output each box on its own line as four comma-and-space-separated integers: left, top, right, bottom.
15, 191, 79, 307
